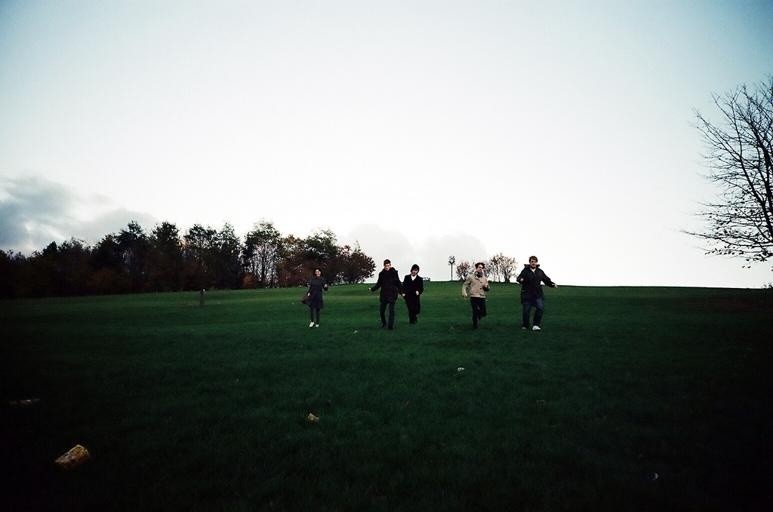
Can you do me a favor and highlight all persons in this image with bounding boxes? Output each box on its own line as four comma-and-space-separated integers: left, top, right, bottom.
461, 263, 490, 328
368, 259, 401, 330
306, 268, 328, 328
402, 264, 424, 325
516, 256, 558, 332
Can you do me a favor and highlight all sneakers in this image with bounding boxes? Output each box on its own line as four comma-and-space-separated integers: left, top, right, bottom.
533, 325, 541, 330
309, 322, 314, 327
316, 324, 319, 327
